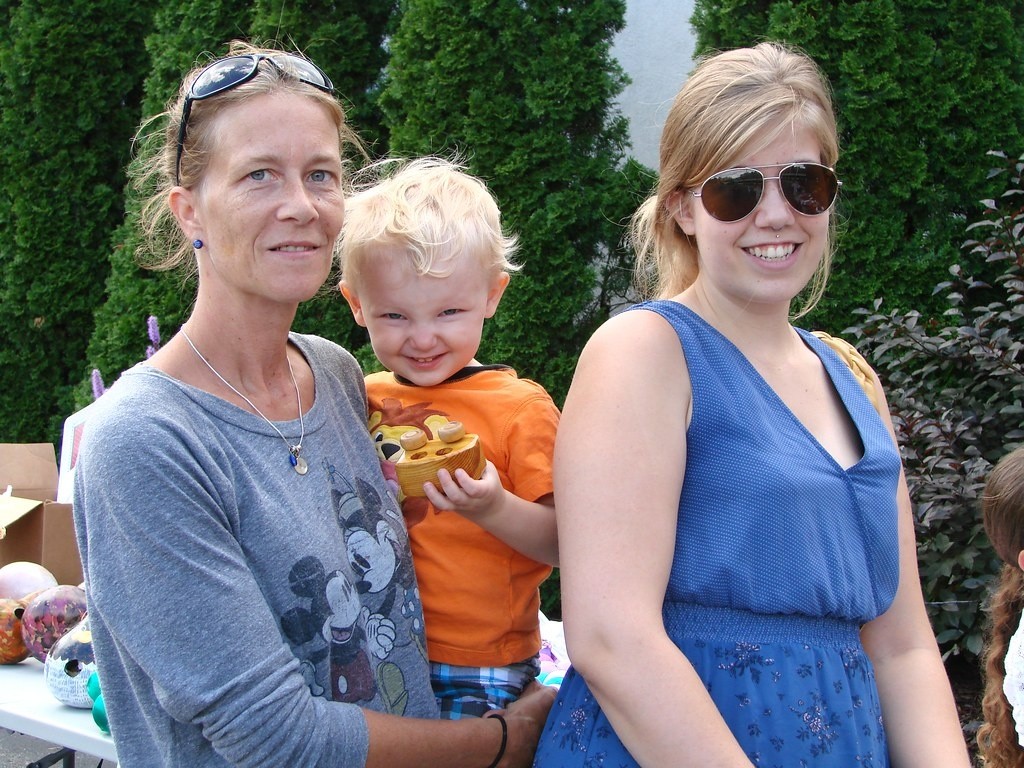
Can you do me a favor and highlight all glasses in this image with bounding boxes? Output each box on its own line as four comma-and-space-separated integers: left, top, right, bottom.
686, 161, 844, 222
175, 53, 346, 184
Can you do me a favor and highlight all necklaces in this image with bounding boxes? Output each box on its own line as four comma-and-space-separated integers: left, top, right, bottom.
181, 326, 309, 476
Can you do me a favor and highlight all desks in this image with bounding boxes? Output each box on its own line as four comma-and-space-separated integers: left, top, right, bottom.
0, 657, 120, 768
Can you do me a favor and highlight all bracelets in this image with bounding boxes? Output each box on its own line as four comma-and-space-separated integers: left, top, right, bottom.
488, 714, 507, 768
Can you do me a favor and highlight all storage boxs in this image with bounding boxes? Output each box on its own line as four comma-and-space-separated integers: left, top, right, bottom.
0, 442, 83, 587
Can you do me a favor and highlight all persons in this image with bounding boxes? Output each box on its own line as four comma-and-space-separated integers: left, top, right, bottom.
529, 41, 970, 768
72, 38, 559, 768
334, 154, 560, 768
977, 445, 1024, 768
